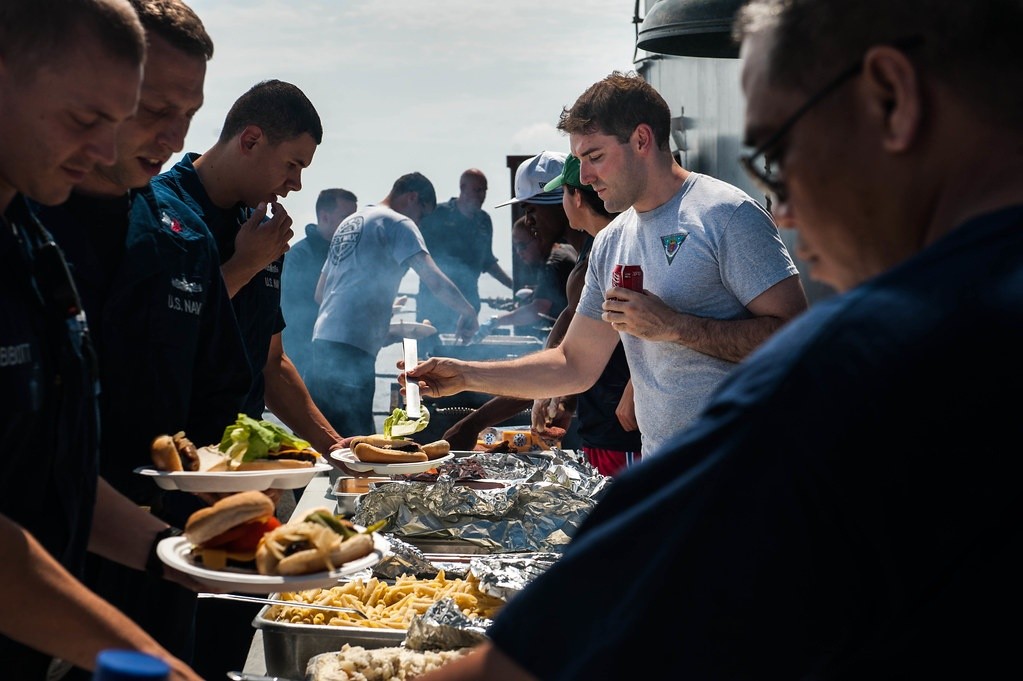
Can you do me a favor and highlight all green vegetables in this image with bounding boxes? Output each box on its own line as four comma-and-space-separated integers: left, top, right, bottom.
220, 414, 311, 462
383, 405, 430, 440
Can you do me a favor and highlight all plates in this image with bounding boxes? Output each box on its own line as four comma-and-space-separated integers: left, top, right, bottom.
331, 447, 454, 476
133, 464, 334, 491
388, 322, 437, 339
157, 526, 389, 593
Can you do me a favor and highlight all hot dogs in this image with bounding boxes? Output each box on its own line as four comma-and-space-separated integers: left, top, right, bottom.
421, 439, 450, 459
228, 448, 317, 471
255, 509, 387, 575
350, 437, 428, 464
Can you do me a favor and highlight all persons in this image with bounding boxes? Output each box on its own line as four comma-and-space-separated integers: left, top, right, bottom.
408, 0, 1023, 681
0, 0, 804, 681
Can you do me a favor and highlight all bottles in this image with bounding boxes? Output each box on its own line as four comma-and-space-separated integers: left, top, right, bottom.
92, 648, 170, 680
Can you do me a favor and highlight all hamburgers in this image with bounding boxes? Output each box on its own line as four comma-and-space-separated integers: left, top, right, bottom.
185, 490, 281, 572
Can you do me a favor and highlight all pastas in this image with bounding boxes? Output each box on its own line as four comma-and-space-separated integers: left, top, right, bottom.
264, 567, 512, 631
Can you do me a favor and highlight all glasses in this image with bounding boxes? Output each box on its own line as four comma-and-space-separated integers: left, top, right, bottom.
512, 238, 534, 251
737, 64, 866, 204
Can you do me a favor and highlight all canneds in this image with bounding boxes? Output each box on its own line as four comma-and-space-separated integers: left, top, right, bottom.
611, 263, 643, 313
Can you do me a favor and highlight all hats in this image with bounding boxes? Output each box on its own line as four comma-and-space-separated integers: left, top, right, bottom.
543, 153, 594, 191
494, 150, 569, 209
